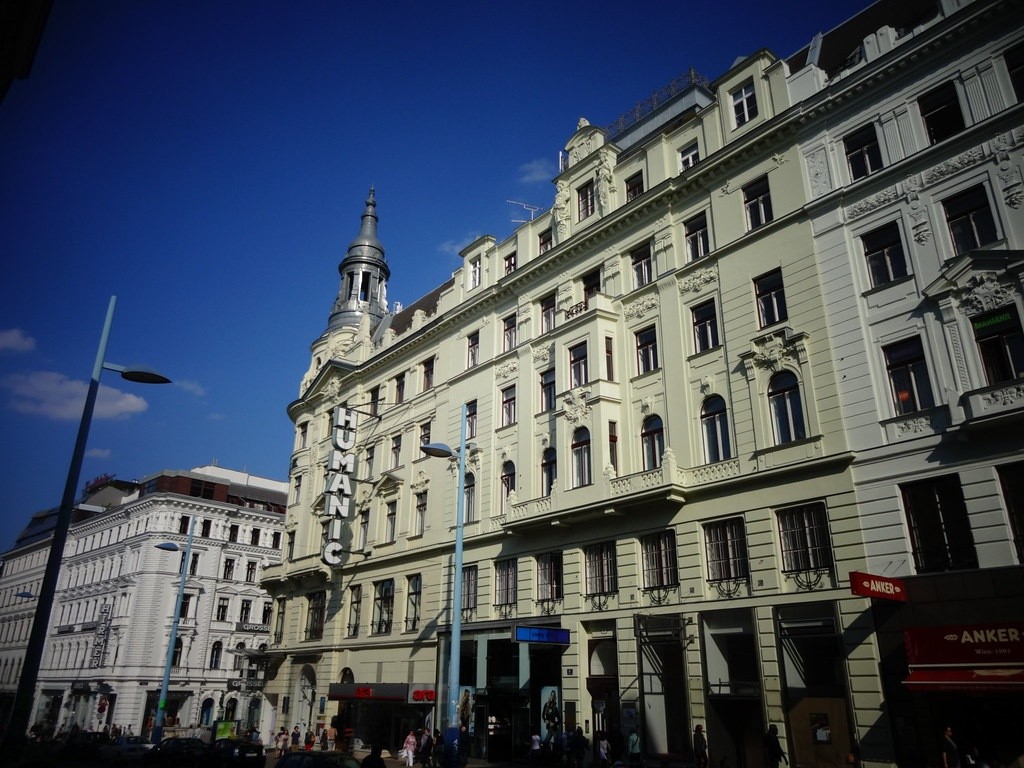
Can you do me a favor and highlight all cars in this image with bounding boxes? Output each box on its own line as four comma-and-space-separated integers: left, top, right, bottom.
196, 738, 266, 768
272, 749, 365, 768
137, 737, 208, 768
97, 736, 156, 761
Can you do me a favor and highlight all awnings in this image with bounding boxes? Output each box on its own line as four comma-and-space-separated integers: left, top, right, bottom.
901, 662, 1024, 695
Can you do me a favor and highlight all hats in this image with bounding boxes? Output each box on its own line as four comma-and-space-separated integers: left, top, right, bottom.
307, 727, 310, 730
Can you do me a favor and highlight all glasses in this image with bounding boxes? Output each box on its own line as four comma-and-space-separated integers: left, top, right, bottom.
948, 730, 951, 732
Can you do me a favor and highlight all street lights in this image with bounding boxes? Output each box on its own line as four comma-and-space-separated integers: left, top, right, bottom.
418, 441, 465, 767
0, 360, 173, 768
151, 541, 189, 746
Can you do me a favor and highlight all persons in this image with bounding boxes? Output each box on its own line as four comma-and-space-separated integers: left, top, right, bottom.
628, 729, 641, 768
403, 726, 471, 768
764, 724, 788, 768
272, 724, 338, 759
30, 722, 134, 743
941, 726, 963, 768
360, 743, 387, 768
693, 725, 708, 768
599, 734, 611, 768
531, 727, 589, 768
460, 689, 471, 731
542, 690, 559, 744
172, 724, 205, 741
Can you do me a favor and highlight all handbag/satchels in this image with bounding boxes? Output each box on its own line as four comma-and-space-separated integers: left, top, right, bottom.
398, 749, 407, 759
277, 739, 283, 749
